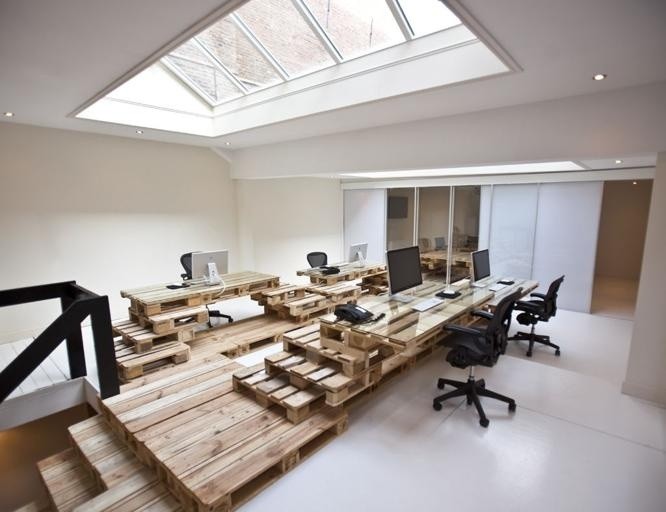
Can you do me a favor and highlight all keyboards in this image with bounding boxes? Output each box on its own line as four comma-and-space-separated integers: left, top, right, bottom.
411, 298, 444, 311
489, 284, 507, 292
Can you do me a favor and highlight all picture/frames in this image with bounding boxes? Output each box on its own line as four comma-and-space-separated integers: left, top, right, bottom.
388, 196, 408, 218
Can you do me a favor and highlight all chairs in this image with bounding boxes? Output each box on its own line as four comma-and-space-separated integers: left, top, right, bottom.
179, 253, 233, 329
507, 276, 565, 356
307, 252, 327, 268
432, 287, 523, 427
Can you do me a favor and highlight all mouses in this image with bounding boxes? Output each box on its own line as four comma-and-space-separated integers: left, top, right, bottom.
501, 279, 510, 283
443, 290, 455, 295
174, 284, 181, 287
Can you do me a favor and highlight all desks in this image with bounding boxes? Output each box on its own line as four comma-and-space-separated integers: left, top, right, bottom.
111, 251, 540, 427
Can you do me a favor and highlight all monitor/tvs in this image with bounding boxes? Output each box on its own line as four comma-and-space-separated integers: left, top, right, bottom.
385, 245, 422, 303
349, 242, 368, 268
471, 249, 492, 288
191, 249, 228, 286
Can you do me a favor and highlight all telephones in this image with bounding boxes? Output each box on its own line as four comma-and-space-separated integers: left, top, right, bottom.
322, 267, 340, 274
334, 303, 373, 324
